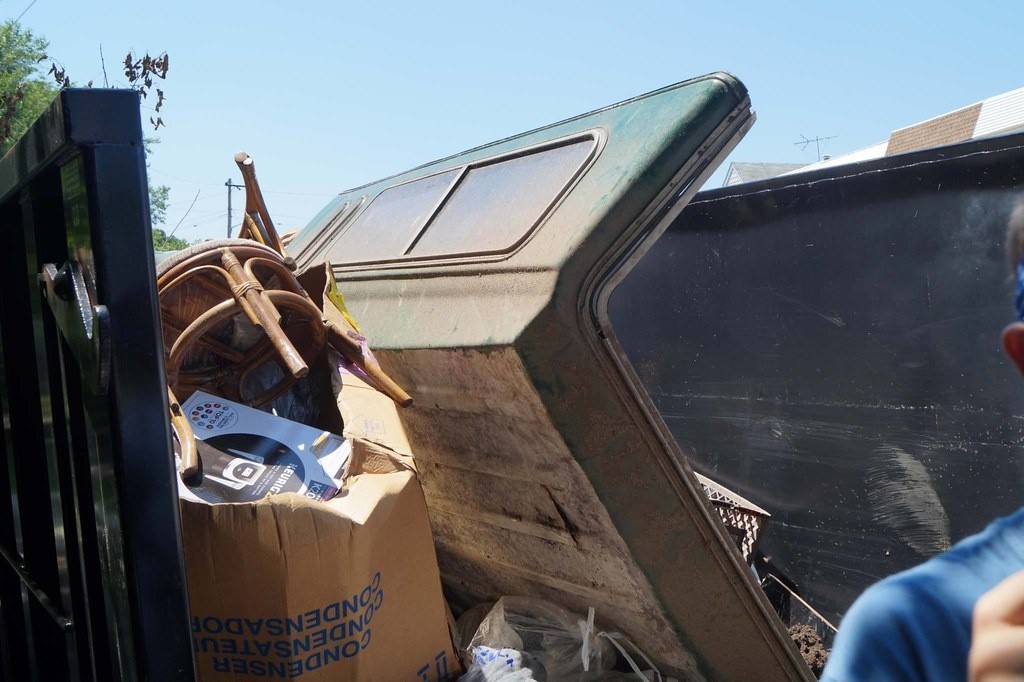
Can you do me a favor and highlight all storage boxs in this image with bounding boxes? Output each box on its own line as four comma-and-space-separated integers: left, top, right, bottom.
694, 471, 771, 568
179, 259, 469, 681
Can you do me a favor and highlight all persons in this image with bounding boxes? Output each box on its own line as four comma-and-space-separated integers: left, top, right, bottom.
820, 191, 1024, 682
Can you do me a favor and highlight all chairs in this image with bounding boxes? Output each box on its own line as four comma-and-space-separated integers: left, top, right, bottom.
154, 152, 412, 481
